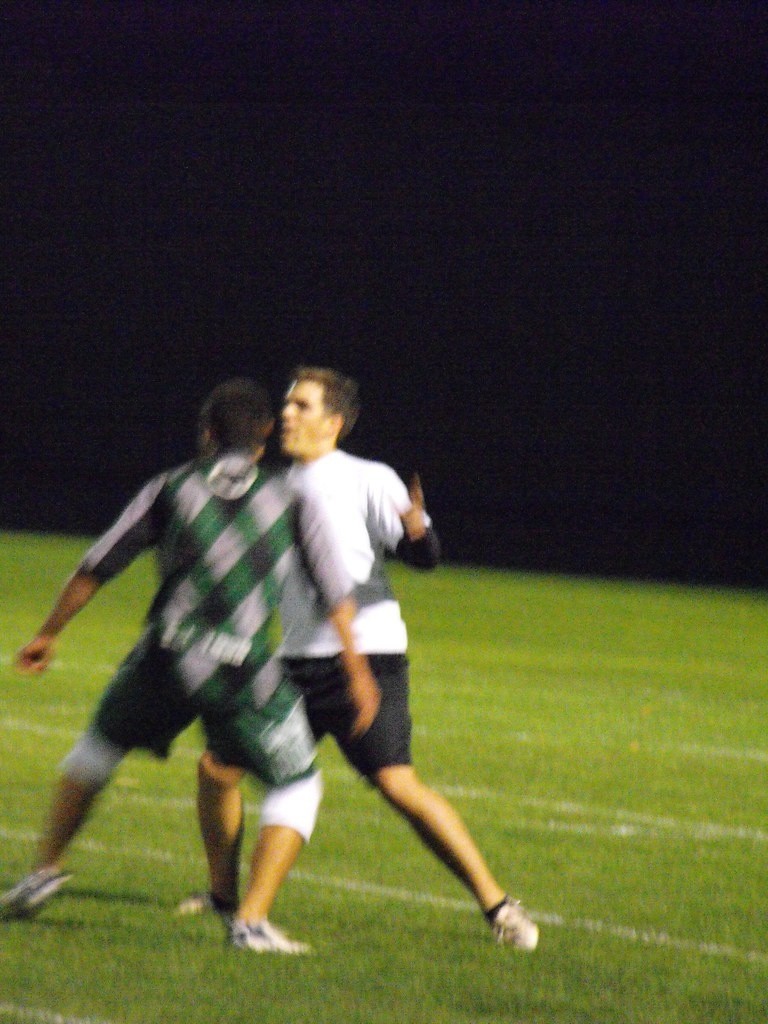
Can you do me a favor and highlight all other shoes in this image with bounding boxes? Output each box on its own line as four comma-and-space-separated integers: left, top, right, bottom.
488, 898, 540, 950
2, 866, 72, 916
228, 918, 309, 954
176, 894, 239, 916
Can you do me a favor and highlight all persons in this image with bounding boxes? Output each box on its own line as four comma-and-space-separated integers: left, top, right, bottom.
174, 365, 539, 952
0, 381, 383, 955
